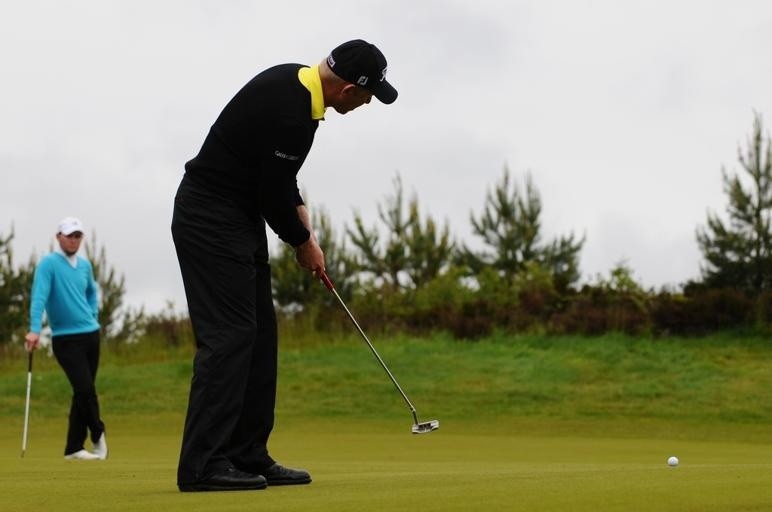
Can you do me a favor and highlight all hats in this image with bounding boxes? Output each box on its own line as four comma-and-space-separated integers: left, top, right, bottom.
56, 217, 85, 237
327, 39, 400, 105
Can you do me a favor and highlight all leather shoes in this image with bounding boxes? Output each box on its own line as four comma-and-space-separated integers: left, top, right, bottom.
175, 459, 268, 491
268, 463, 312, 485
64, 449, 101, 459
92, 431, 107, 459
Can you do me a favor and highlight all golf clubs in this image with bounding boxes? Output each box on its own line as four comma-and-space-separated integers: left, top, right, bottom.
320, 270, 439, 433
21, 348, 34, 456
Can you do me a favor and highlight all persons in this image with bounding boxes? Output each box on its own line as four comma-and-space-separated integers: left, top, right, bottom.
23, 215, 109, 460
170, 39, 398, 491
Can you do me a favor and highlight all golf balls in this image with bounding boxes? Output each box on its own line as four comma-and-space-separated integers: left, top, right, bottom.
668, 457, 678, 466
